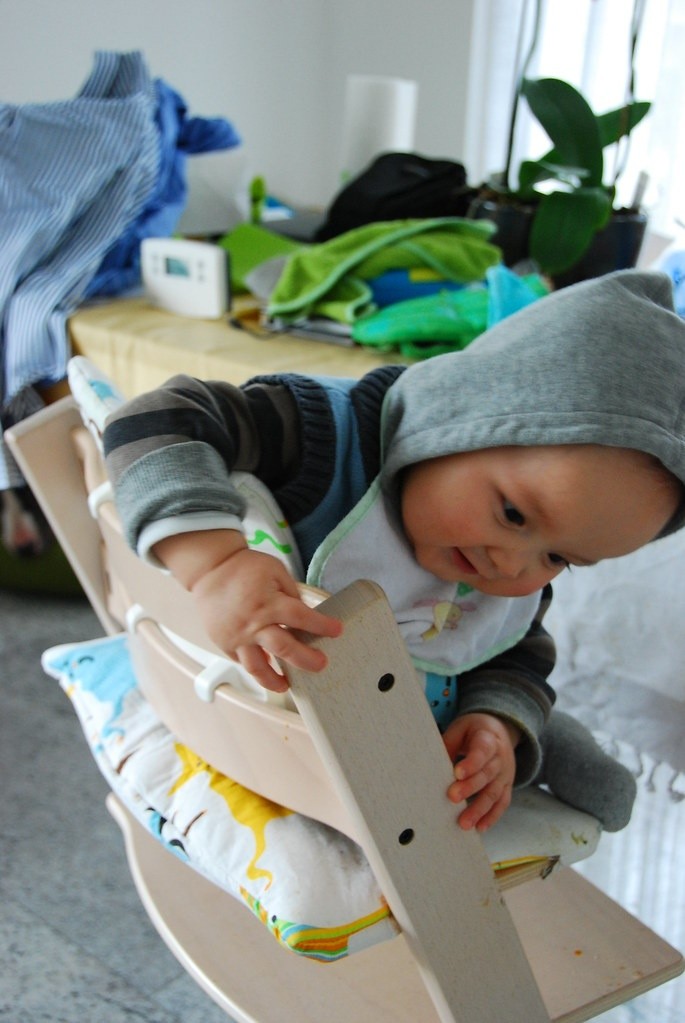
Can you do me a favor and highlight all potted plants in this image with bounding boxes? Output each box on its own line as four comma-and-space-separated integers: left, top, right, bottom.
476, 77, 652, 298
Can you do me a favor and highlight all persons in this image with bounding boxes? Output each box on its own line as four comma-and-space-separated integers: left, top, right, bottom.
99, 267, 685, 833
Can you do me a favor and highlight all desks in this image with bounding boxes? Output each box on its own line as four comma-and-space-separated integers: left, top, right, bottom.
65, 289, 416, 425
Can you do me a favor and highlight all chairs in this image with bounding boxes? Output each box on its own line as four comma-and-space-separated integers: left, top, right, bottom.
3, 391, 682, 1023
